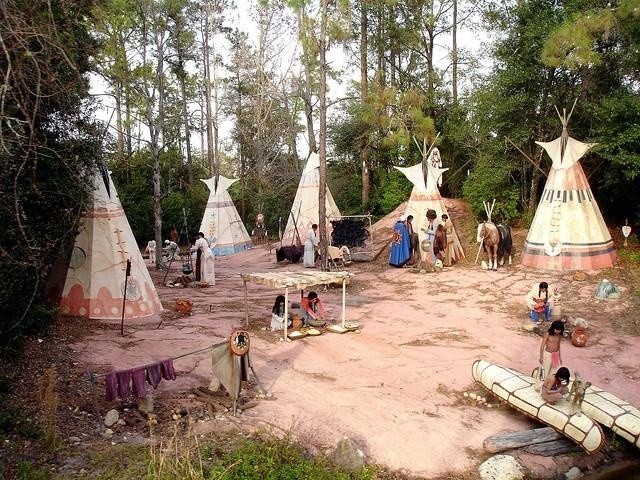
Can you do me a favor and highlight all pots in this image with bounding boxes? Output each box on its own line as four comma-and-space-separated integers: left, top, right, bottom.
182, 263, 192, 275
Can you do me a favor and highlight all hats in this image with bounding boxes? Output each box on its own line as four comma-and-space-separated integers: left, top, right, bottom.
398, 209, 449, 221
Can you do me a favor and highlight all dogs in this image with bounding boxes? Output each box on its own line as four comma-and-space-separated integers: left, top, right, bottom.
566, 372, 592, 418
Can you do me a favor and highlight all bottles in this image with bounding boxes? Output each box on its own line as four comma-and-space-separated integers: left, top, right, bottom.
571, 327, 588, 348
292, 315, 303, 331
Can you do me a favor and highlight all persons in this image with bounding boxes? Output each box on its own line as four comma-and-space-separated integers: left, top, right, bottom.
539, 321, 565, 381
299, 292, 326, 328
271, 296, 292, 331
390, 208, 454, 271
527, 282, 554, 322
303, 224, 319, 268
541, 367, 571, 404
145, 232, 215, 286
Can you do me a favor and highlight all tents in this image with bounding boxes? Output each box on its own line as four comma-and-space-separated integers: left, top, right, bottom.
520, 137, 618, 271
281, 152, 342, 246
392, 160, 466, 267
42, 163, 165, 319
198, 175, 255, 256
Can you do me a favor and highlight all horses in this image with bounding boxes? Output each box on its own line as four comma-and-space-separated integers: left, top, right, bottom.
474, 218, 514, 272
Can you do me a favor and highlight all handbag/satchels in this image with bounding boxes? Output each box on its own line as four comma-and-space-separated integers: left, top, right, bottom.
421, 239, 431, 252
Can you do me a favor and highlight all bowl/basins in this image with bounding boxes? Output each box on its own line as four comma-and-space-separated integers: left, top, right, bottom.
308, 320, 327, 327
